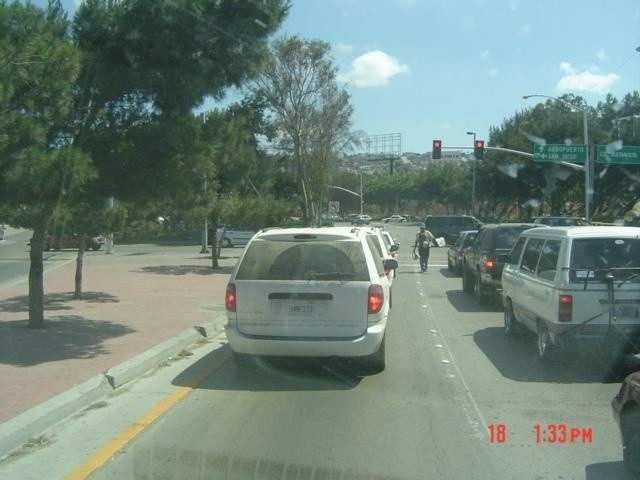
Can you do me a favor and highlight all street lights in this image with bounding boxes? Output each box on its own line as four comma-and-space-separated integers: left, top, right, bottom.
466, 131, 476, 215
522, 94, 591, 218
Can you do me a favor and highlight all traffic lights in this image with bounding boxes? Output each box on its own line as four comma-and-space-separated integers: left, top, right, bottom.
431, 140, 442, 159
474, 140, 486, 161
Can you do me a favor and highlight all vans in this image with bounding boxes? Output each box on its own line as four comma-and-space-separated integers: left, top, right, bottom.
423, 213, 640, 362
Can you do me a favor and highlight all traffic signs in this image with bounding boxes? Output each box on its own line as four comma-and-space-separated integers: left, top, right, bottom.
532, 142, 588, 163
596, 143, 639, 166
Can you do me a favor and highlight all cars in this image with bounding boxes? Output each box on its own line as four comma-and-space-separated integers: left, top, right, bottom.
219, 224, 400, 370
321, 213, 421, 225
29, 230, 105, 250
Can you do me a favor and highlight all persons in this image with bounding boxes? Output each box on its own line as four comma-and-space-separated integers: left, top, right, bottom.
413, 225, 441, 272
